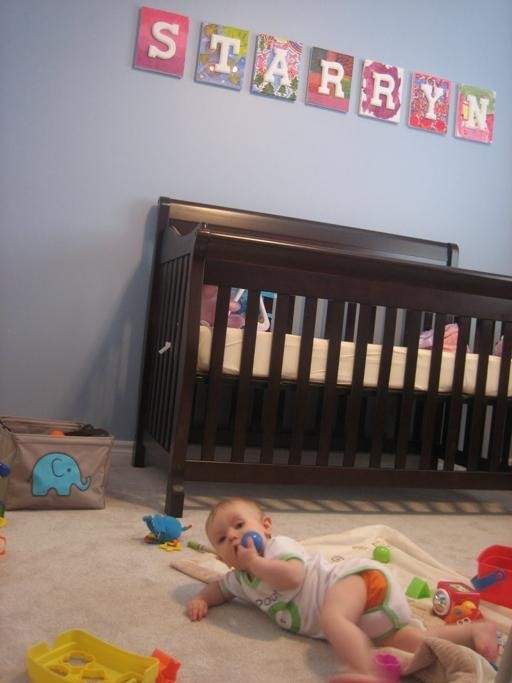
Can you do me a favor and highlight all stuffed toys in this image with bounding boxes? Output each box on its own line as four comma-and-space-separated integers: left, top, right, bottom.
142, 513, 192, 552
201, 284, 244, 328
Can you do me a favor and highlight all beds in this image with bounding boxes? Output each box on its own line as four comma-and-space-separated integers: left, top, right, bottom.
133, 195, 512, 518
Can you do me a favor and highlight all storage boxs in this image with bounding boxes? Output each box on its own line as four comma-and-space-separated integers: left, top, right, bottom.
0, 414, 114, 512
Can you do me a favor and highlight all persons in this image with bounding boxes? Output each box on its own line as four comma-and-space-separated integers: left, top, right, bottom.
184, 495, 499, 682
